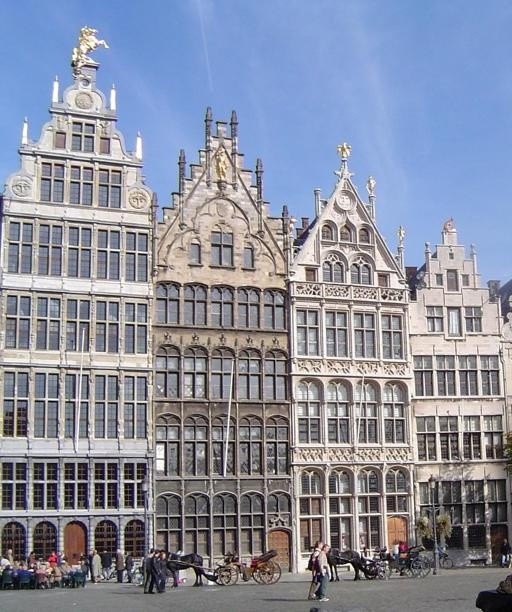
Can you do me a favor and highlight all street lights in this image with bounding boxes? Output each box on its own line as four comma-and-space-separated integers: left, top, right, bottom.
141, 477, 152, 584
428, 475, 439, 575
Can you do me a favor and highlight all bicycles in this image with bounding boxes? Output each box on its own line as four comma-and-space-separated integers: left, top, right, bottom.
431, 553, 454, 568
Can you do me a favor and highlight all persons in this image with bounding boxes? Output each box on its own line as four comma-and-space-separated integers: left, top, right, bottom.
0, 549, 168, 594
393, 541, 408, 576
315, 544, 330, 601
216, 151, 227, 179
361, 547, 368, 560
500, 538, 511, 568
79, 26, 96, 51
433, 543, 444, 560
308, 541, 323, 600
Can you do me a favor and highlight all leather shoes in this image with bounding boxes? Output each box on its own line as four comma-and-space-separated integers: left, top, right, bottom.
144, 589, 166, 594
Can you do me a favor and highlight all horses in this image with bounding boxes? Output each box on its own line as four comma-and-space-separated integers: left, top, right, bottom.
325, 547, 363, 582
160, 549, 203, 588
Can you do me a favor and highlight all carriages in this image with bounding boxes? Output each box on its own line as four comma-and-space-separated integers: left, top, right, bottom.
312, 545, 431, 579
159, 551, 281, 587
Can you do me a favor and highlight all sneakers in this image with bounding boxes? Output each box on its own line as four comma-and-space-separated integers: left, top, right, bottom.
313, 594, 329, 602
